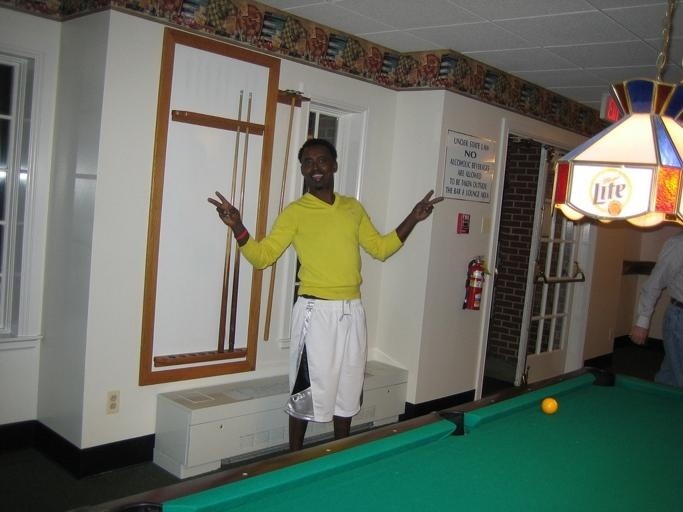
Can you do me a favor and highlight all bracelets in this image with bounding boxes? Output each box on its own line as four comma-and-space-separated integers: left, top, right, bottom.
234, 228, 246, 241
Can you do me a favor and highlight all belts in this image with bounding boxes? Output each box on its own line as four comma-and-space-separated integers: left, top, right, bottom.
670, 297, 683, 308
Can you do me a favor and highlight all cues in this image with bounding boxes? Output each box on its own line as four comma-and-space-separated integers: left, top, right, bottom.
264, 90, 296, 340
218, 90, 252, 353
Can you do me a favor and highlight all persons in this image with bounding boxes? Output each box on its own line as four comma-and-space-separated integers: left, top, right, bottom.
206, 139, 443, 451
630, 229, 682, 390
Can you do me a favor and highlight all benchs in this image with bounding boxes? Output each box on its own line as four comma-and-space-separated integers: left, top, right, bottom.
148, 359, 406, 479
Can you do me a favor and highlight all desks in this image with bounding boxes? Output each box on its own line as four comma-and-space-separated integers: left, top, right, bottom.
70, 364, 681, 512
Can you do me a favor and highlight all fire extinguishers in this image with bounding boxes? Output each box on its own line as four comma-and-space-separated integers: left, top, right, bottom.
462, 256, 486, 310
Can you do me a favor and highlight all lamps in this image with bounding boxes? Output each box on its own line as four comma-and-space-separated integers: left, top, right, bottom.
549, 1, 682, 230
